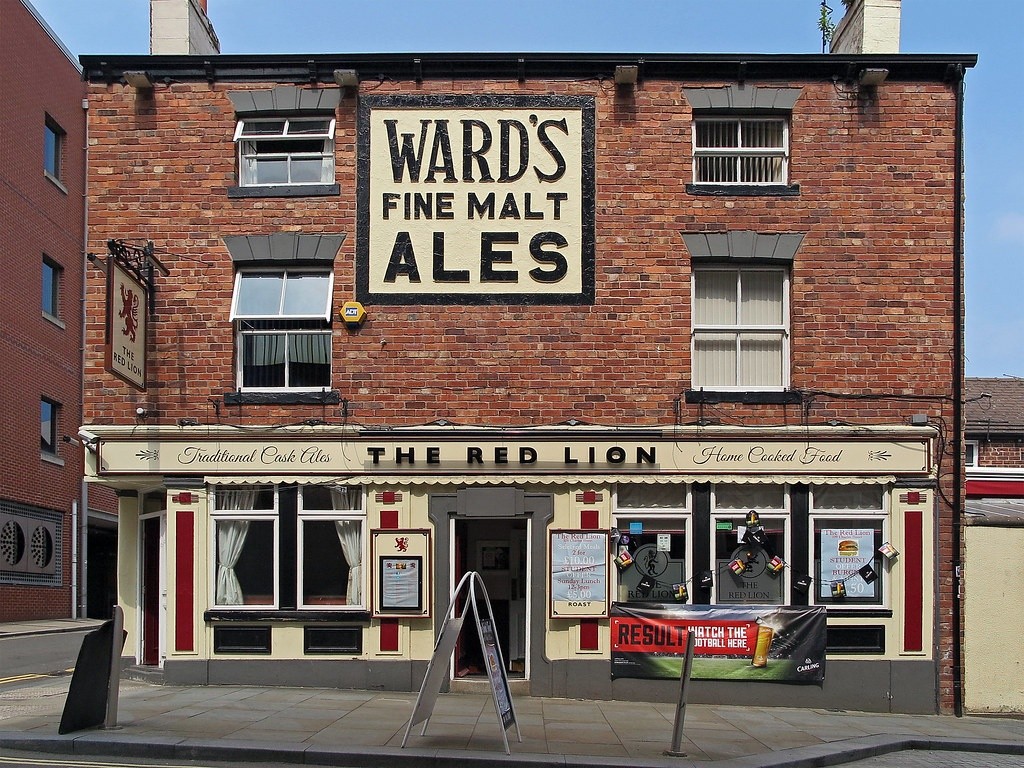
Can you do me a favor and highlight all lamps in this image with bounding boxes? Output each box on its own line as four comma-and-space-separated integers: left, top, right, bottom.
859, 68, 889, 86
123, 70, 154, 88
333, 69, 361, 86
615, 66, 638, 85
